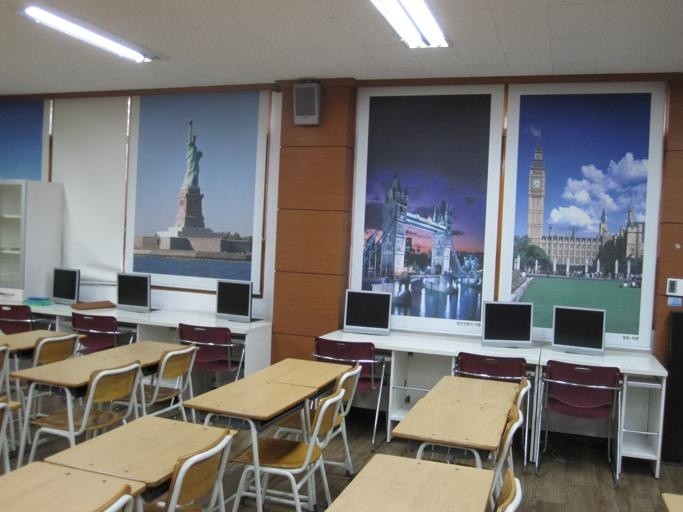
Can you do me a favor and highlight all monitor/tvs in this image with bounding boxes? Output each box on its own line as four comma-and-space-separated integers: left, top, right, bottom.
52, 267, 81, 306
343, 288, 392, 336
215, 278, 254, 323
481, 300, 534, 348
552, 304, 607, 357
116, 273, 152, 313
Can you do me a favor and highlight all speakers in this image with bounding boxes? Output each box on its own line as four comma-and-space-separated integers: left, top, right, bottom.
291, 82, 321, 127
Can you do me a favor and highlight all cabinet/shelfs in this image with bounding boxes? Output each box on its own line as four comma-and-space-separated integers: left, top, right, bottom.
0, 179, 64, 304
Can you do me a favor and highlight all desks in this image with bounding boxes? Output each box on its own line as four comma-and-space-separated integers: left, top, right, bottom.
534, 343, 669, 479
